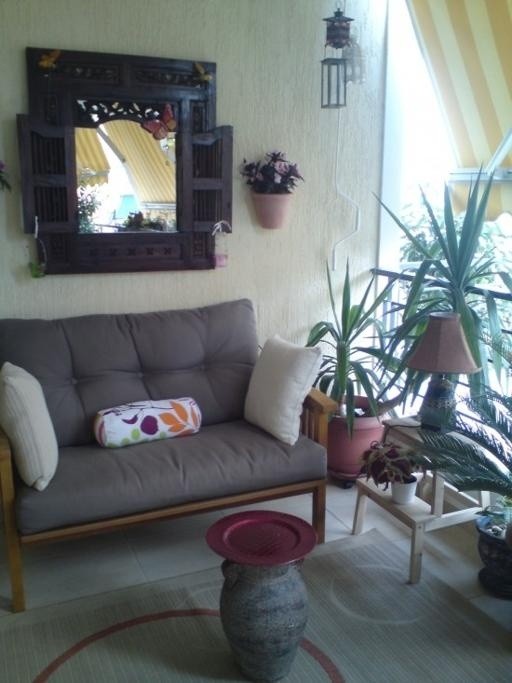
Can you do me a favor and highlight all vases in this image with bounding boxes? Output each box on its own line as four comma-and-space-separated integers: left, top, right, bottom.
253, 192, 293, 232
389, 477, 419, 506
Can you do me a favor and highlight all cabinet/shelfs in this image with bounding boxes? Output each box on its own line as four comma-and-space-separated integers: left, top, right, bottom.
347, 415, 495, 583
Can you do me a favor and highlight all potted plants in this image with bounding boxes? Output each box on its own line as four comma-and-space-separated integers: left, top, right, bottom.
420, 378, 511, 601
304, 129, 511, 487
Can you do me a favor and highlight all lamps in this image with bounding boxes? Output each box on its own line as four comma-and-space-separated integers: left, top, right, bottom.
404, 306, 479, 432
318, 0, 368, 111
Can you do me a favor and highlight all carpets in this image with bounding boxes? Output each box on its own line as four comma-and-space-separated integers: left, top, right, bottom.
0, 526, 512, 683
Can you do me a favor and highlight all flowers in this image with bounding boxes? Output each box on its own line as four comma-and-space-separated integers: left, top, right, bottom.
240, 145, 307, 194
356, 439, 420, 492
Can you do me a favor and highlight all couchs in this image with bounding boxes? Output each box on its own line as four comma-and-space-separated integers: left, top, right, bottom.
0, 296, 338, 616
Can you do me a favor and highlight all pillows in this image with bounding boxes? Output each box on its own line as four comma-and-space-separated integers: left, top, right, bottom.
242, 330, 324, 445
0, 355, 61, 493
93, 397, 206, 450
